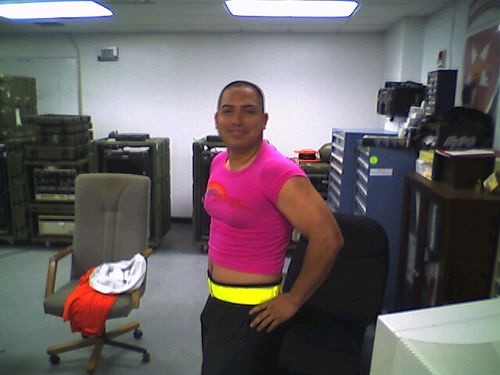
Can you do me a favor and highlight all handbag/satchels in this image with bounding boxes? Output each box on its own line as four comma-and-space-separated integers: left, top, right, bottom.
88, 253, 146, 293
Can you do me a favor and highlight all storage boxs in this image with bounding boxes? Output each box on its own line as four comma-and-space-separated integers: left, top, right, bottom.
1, 75, 97, 246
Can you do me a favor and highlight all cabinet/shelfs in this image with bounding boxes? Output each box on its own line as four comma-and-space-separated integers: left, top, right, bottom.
193, 136, 228, 254
327, 127, 399, 213
352, 143, 416, 313
97, 133, 171, 249
391, 172, 500, 318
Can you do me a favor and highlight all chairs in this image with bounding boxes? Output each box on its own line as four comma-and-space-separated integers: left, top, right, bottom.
42, 174, 152, 373
271, 213, 389, 375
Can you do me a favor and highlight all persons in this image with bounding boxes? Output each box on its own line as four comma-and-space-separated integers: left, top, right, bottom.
200, 80, 344, 375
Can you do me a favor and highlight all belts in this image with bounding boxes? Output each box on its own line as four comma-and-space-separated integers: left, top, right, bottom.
207, 278, 282, 305
